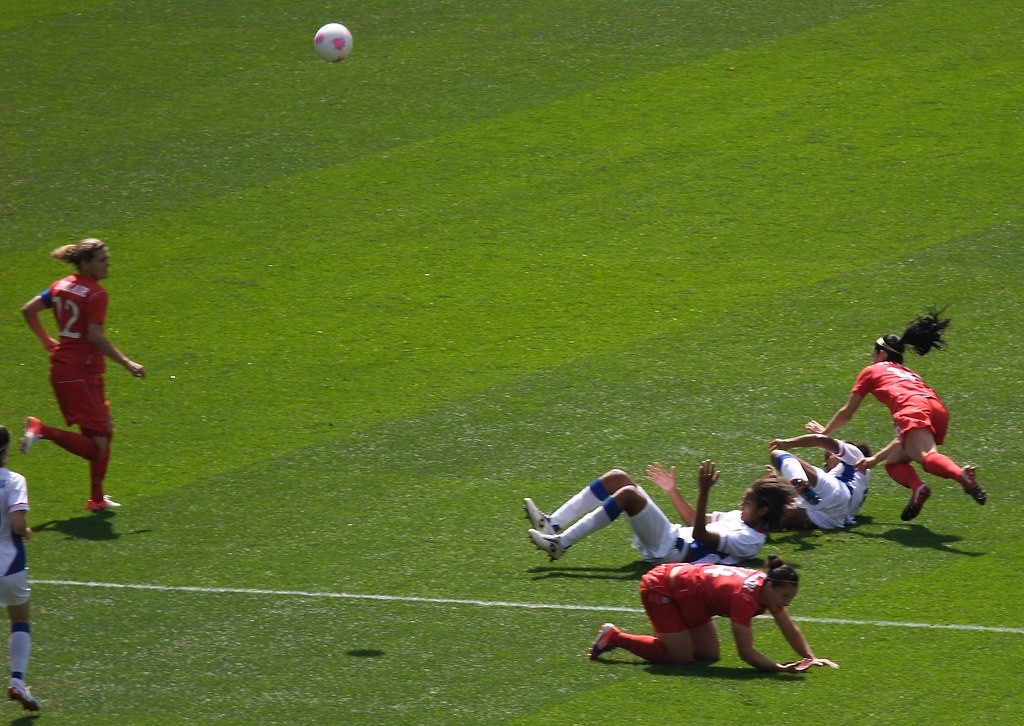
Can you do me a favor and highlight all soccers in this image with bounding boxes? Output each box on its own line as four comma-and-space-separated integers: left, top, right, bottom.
310, 21, 355, 63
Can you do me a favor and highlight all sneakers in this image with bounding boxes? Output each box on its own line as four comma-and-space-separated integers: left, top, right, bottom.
523, 497, 553, 536
527, 528, 572, 561
87, 493, 121, 510
9, 679, 40, 711
587, 622, 621, 660
19, 416, 41, 453
957, 465, 987, 505
900, 483, 931, 521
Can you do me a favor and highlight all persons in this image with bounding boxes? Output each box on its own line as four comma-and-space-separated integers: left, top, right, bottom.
0, 425, 41, 711
520, 304, 989, 567
19, 236, 148, 512
587, 554, 841, 673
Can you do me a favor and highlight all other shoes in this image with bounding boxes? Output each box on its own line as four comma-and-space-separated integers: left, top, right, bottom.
790, 477, 820, 505
